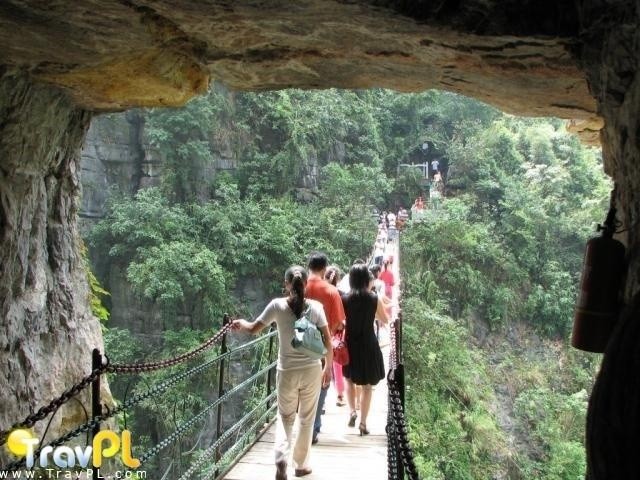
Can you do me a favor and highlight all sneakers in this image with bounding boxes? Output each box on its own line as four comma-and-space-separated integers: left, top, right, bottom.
295, 467, 311, 477
312, 438, 318, 444
276, 462, 287, 480
336, 398, 345, 406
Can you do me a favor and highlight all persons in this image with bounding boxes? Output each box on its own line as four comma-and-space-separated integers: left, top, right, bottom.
235, 141, 444, 479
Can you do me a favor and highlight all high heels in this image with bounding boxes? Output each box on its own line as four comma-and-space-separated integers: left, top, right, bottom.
348, 411, 357, 426
359, 423, 369, 436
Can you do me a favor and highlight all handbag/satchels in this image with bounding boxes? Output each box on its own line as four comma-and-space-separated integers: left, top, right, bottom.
291, 317, 327, 359
331, 337, 350, 366
